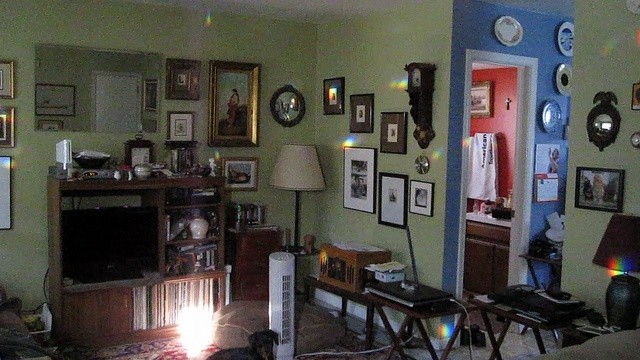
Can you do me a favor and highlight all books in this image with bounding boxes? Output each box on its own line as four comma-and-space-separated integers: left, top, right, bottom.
171, 217, 191, 240
132, 278, 221, 329
165, 213, 172, 240
167, 244, 218, 273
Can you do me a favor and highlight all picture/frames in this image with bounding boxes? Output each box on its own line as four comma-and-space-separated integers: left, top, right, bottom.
222, 157, 258, 191
143, 79, 157, 111
379, 171, 408, 229
471, 80, 493, 119
0, 60, 15, 99
410, 179, 433, 217
208, 60, 261, 147
0, 156, 11, 229
632, 83, 640, 111
167, 110, 193, 141
350, 93, 373, 133
166, 57, 201, 100
323, 77, 345, 115
344, 146, 377, 214
36, 83, 76, 116
39, 120, 63, 130
0, 106, 15, 148
380, 112, 407, 154
575, 167, 624, 213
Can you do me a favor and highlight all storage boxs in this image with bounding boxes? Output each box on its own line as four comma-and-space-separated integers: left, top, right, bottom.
319, 244, 391, 295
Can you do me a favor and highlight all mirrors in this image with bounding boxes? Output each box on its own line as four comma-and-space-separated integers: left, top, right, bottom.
270, 83, 306, 127
34, 42, 160, 133
587, 91, 620, 152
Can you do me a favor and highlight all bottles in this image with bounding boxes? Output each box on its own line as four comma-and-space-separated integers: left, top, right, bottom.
496, 198, 504, 207
485, 200, 491, 215
507, 189, 512, 208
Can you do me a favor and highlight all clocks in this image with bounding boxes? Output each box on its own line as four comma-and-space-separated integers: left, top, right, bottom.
403, 62, 435, 148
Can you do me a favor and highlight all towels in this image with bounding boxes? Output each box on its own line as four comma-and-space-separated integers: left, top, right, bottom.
468, 132, 499, 201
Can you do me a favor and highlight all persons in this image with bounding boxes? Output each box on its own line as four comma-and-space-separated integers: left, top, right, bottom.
227, 89, 240, 128
548, 147, 559, 173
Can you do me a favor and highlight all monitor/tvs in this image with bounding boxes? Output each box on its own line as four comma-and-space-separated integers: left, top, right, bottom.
60, 205, 159, 284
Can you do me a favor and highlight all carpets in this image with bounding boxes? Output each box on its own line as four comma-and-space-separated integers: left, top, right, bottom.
462, 290, 506, 334
93, 329, 416, 360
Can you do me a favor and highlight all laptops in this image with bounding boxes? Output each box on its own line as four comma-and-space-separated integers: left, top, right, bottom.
368, 280, 454, 310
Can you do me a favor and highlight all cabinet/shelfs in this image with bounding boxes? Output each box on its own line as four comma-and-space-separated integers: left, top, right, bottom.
464, 220, 510, 295
228, 227, 278, 301
48, 175, 227, 346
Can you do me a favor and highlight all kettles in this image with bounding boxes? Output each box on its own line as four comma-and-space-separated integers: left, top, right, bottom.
247, 204, 258, 226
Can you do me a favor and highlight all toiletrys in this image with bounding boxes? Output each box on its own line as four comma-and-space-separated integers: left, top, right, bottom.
506, 189, 513, 208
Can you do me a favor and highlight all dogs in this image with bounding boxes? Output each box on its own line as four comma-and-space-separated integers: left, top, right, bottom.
206, 330, 279, 360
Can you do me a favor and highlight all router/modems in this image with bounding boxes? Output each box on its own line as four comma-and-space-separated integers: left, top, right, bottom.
55, 139, 72, 170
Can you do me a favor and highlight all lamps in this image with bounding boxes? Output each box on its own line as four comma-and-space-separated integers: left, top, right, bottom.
270, 144, 326, 251
592, 213, 640, 330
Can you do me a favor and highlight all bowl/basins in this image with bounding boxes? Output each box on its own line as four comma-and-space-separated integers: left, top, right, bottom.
135, 171, 152, 181
135, 165, 151, 171
74, 158, 111, 169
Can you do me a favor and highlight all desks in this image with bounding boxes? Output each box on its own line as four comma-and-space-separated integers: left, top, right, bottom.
368, 293, 476, 360
563, 324, 597, 348
469, 294, 572, 360
282, 246, 319, 293
519, 254, 561, 344
305, 277, 368, 354
